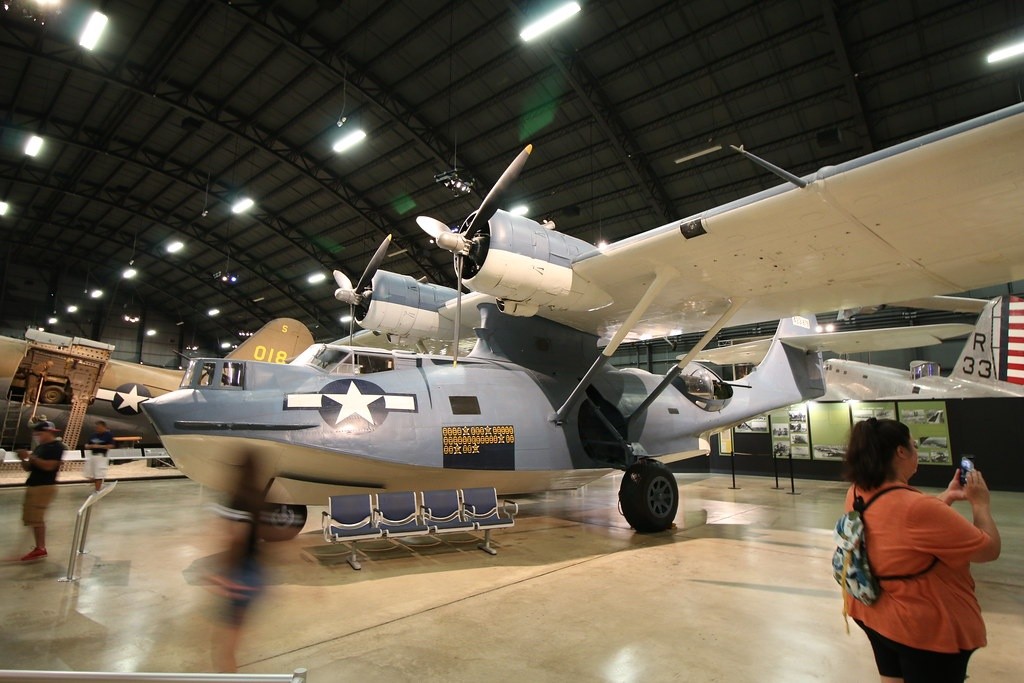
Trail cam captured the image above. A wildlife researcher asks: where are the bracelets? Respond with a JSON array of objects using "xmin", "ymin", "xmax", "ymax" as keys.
[{"xmin": 23, "ymin": 458, "xmax": 30, "ymax": 463}]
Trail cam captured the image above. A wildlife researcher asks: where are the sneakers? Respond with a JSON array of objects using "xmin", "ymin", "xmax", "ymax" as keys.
[{"xmin": 21, "ymin": 546, "xmax": 47, "ymax": 561}]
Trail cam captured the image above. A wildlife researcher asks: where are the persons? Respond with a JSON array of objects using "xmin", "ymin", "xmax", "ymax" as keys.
[
  {"xmin": 845, "ymin": 419, "xmax": 1002, "ymax": 683},
  {"xmin": 18, "ymin": 414, "xmax": 63, "ymax": 562},
  {"xmin": 85, "ymin": 419, "xmax": 113, "ymax": 493}
]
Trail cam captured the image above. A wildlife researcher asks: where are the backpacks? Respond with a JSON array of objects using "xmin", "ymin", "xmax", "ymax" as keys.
[{"xmin": 832, "ymin": 483, "xmax": 939, "ymax": 635}]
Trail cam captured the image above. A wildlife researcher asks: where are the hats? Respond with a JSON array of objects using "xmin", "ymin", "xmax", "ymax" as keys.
[
  {"xmin": 32, "ymin": 421, "xmax": 60, "ymax": 431},
  {"xmin": 38, "ymin": 414, "xmax": 47, "ymax": 420}
]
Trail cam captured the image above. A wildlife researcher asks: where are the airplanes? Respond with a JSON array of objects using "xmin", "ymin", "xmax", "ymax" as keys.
[
  {"xmin": 140, "ymin": 102, "xmax": 1024, "ymax": 535},
  {"xmin": 823, "ymin": 295, "xmax": 1024, "ymax": 401},
  {"xmin": 0, "ymin": 318, "xmax": 315, "ymax": 462}
]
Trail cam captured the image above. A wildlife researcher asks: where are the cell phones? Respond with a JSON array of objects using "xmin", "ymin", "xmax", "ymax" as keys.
[{"xmin": 960, "ymin": 457, "xmax": 974, "ymax": 486}]
